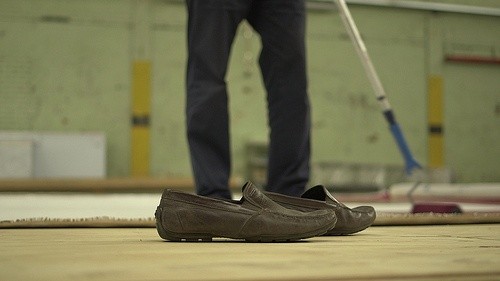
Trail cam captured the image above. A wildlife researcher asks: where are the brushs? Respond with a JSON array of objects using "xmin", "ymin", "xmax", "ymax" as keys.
[{"xmin": 335, "ymin": 0, "xmax": 500, "ymax": 203}]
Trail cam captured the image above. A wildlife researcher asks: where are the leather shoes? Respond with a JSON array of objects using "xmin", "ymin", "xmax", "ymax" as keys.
[
  {"xmin": 155, "ymin": 180, "xmax": 338, "ymax": 243},
  {"xmin": 263, "ymin": 185, "xmax": 376, "ymax": 236}
]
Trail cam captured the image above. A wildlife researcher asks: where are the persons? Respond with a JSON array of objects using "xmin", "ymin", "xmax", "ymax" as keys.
[{"xmin": 183, "ymin": 0, "xmax": 323, "ymax": 208}]
